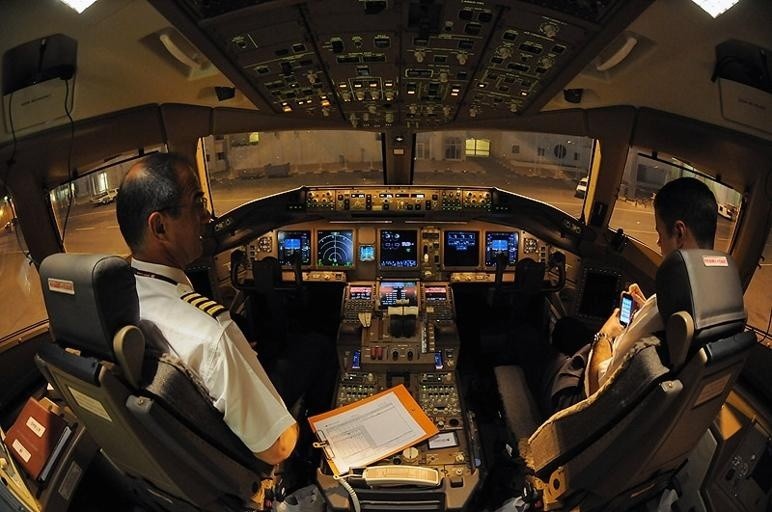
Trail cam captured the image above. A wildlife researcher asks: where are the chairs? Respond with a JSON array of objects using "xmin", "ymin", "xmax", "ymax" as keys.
[
  {"xmin": 25, "ymin": 244, "xmax": 302, "ymax": 507},
  {"xmin": 486, "ymin": 244, "xmax": 762, "ymax": 512}
]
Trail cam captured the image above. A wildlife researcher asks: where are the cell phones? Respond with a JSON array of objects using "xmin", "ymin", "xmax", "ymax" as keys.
[{"xmin": 620, "ymin": 293, "xmax": 633, "ymax": 327}]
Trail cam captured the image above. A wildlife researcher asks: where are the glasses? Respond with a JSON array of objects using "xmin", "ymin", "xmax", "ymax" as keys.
[{"xmin": 146, "ymin": 197, "xmax": 208, "ymax": 226}]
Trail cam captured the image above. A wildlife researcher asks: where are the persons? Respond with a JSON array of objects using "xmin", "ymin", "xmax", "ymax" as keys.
[
  {"xmin": 534, "ymin": 176, "xmax": 719, "ymax": 423},
  {"xmin": 115, "ymin": 151, "xmax": 301, "ymax": 466}
]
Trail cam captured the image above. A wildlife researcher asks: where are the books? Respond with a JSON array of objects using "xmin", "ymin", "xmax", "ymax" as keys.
[{"xmin": 4, "ymin": 397, "xmax": 79, "ymax": 490}]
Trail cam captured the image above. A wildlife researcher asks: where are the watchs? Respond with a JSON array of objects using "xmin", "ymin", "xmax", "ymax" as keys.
[{"xmin": 590, "ymin": 332, "xmax": 614, "ymax": 357}]
